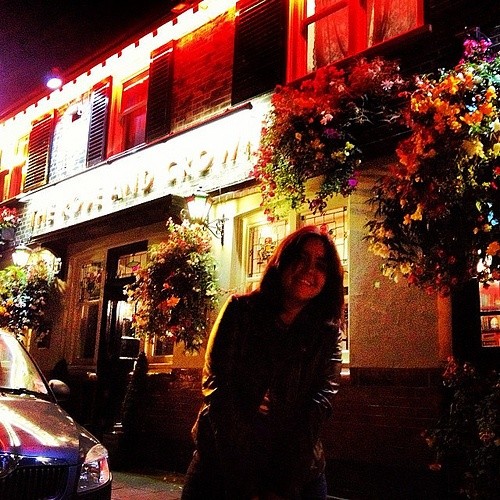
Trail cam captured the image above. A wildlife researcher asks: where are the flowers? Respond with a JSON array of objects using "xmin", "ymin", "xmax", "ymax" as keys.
[
  {"xmin": 121, "ymin": 214, "xmax": 224, "ymax": 353},
  {"xmin": 0, "ymin": 262, "xmax": 56, "ymax": 334},
  {"xmin": 249, "ymin": 37, "xmax": 500, "ymax": 297}
]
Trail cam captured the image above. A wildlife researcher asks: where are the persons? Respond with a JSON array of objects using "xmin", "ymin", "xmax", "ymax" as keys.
[{"xmin": 180, "ymin": 224, "xmax": 344, "ymax": 500}]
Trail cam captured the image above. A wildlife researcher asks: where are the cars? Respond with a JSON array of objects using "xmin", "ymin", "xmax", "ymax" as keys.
[{"xmin": 0, "ymin": 328, "xmax": 115, "ymax": 499}]
